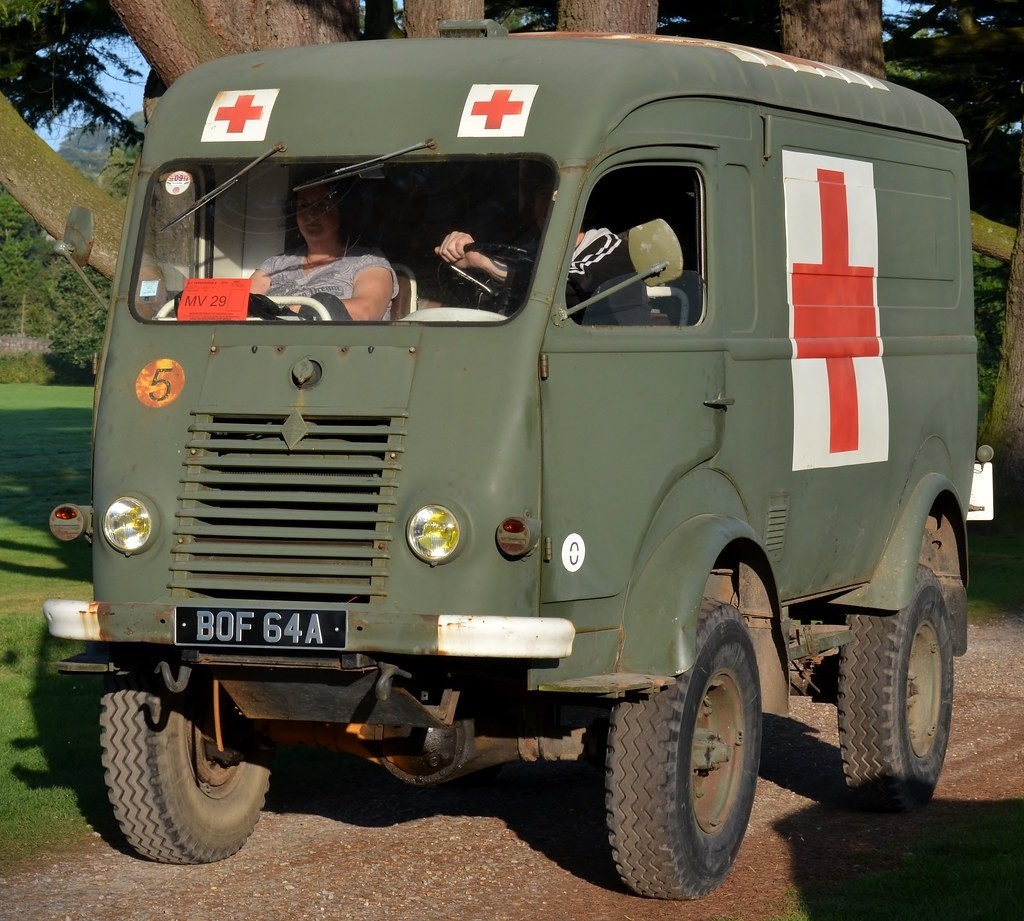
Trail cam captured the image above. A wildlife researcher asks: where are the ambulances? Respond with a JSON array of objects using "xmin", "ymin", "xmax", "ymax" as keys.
[{"xmin": 37, "ymin": 30, "xmax": 996, "ymax": 902}]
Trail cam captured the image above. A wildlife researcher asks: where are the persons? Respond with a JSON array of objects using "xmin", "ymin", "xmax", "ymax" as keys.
[
  {"xmin": 246, "ymin": 174, "xmax": 399, "ymax": 323},
  {"xmin": 435, "ymin": 175, "xmax": 649, "ymax": 327}
]
